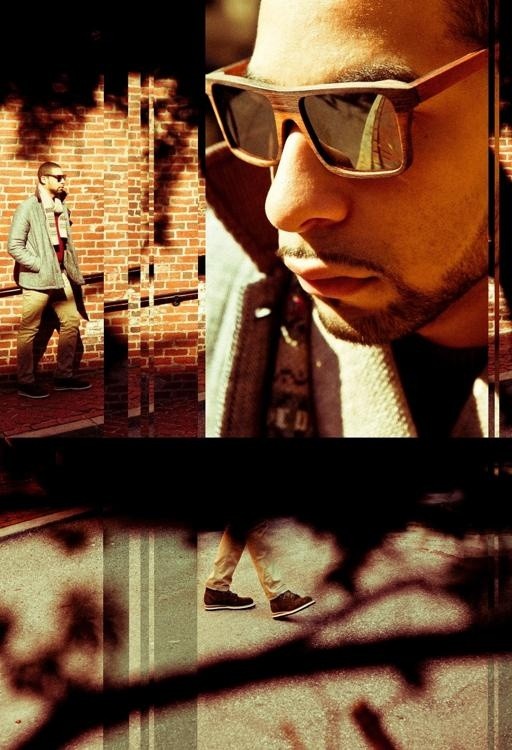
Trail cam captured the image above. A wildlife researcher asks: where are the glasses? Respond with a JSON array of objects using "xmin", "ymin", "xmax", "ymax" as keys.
[
  {"xmin": 204, "ymin": 44, "xmax": 488, "ymax": 184},
  {"xmin": 43, "ymin": 173, "xmax": 68, "ymax": 183}
]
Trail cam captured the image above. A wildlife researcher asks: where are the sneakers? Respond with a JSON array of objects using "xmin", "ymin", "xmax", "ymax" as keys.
[
  {"xmin": 53, "ymin": 379, "xmax": 92, "ymax": 391},
  {"xmin": 269, "ymin": 588, "xmax": 318, "ymax": 620},
  {"xmin": 204, "ymin": 585, "xmax": 258, "ymax": 611},
  {"xmin": 16, "ymin": 379, "xmax": 50, "ymax": 399}
]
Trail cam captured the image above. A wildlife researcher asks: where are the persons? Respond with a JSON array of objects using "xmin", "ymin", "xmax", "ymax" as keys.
[
  {"xmin": 7, "ymin": 161, "xmax": 93, "ymax": 399},
  {"xmin": 206, "ymin": 0, "xmax": 499, "ymax": 440},
  {"xmin": 201, "ymin": 522, "xmax": 316, "ymax": 619}
]
